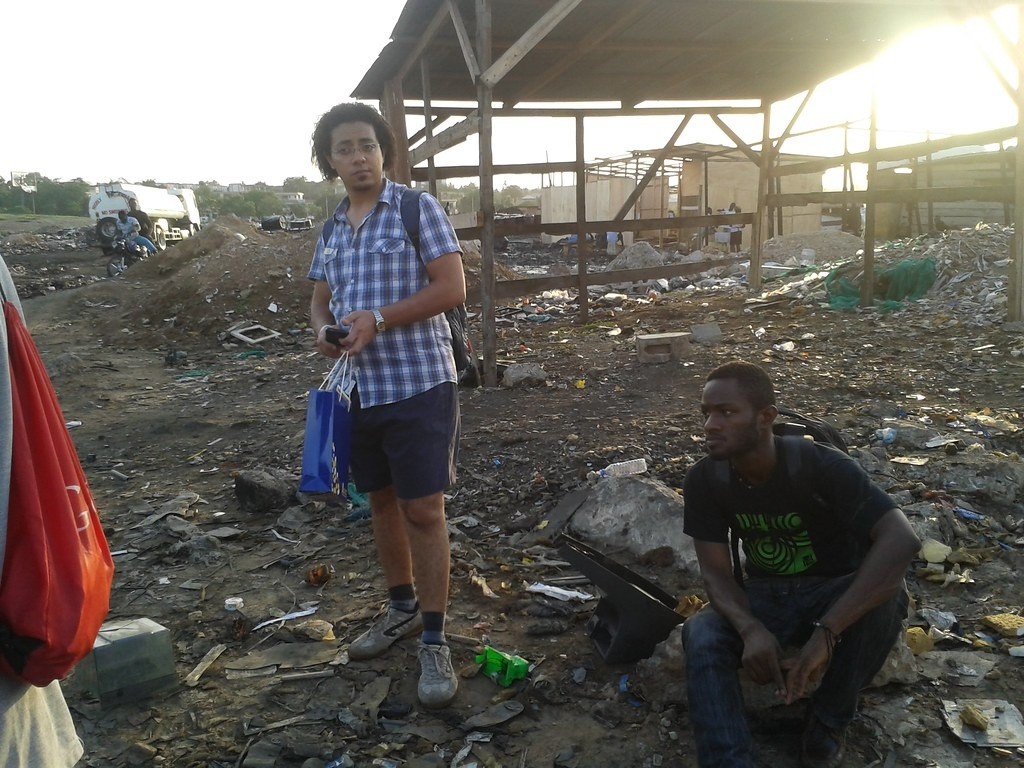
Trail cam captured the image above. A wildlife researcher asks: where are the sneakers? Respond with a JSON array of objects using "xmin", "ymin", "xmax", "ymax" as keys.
[
  {"xmin": 348, "ymin": 601, "xmax": 423, "ymax": 661},
  {"xmin": 416, "ymin": 639, "xmax": 458, "ymax": 709}
]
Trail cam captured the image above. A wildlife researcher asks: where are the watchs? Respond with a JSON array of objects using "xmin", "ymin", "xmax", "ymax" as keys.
[{"xmin": 373, "ymin": 309, "xmax": 386, "ymax": 331}]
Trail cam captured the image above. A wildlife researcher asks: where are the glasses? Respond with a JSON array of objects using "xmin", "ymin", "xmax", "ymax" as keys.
[{"xmin": 328, "ymin": 142, "xmax": 381, "ymax": 161}]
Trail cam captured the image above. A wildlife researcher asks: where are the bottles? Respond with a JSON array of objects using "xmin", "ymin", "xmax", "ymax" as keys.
[{"xmin": 600, "ymin": 458, "xmax": 647, "ymax": 477}]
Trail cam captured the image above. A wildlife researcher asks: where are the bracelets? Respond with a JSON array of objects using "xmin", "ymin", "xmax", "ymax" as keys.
[{"xmin": 813, "ymin": 620, "xmax": 842, "ymax": 661}]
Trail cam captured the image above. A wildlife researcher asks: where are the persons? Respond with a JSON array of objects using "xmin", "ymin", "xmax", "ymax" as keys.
[
  {"xmin": 305, "ymin": 102, "xmax": 469, "ymax": 708},
  {"xmin": 117, "ymin": 211, "xmax": 156, "ymax": 255},
  {"xmin": 127, "ymin": 198, "xmax": 163, "ymax": 251},
  {"xmin": 682, "ymin": 362, "xmax": 923, "ymax": 767},
  {"xmin": 706, "ymin": 201, "xmax": 744, "ymax": 253},
  {"xmin": 0, "ymin": 249, "xmax": 86, "ymax": 768}
]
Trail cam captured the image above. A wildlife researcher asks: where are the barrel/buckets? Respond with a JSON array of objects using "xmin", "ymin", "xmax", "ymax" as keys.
[{"xmin": 801, "ymin": 249, "xmax": 815, "ymax": 265}]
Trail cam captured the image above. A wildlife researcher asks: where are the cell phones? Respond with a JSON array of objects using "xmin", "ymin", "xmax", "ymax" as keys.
[{"xmin": 326, "ymin": 327, "xmax": 350, "ymax": 346}]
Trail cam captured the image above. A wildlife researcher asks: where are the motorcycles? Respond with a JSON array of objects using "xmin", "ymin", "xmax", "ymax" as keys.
[{"xmin": 106, "ymin": 236, "xmax": 142, "ymax": 276}]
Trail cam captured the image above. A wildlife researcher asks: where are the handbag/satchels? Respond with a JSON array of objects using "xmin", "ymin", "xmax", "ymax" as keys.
[
  {"xmin": 3, "ymin": 300, "xmax": 115, "ymax": 687},
  {"xmin": 298, "ymin": 349, "xmax": 352, "ymax": 503}
]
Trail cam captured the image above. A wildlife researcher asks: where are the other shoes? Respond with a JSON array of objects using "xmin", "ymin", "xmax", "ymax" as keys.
[{"xmin": 801, "ymin": 702, "xmax": 845, "ymax": 768}]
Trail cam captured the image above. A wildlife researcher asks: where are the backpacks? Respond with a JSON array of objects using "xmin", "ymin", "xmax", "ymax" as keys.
[
  {"xmin": 323, "ymin": 188, "xmax": 473, "ymax": 381},
  {"xmin": 702, "ymin": 409, "xmax": 858, "ymax": 576}
]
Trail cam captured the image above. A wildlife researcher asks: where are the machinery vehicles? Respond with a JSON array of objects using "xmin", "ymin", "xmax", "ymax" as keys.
[{"xmin": 87, "ymin": 181, "xmax": 202, "ymax": 252}]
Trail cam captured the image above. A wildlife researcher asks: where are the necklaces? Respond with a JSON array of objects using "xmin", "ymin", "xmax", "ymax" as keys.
[{"xmin": 731, "ymin": 465, "xmax": 753, "ymax": 490}]
